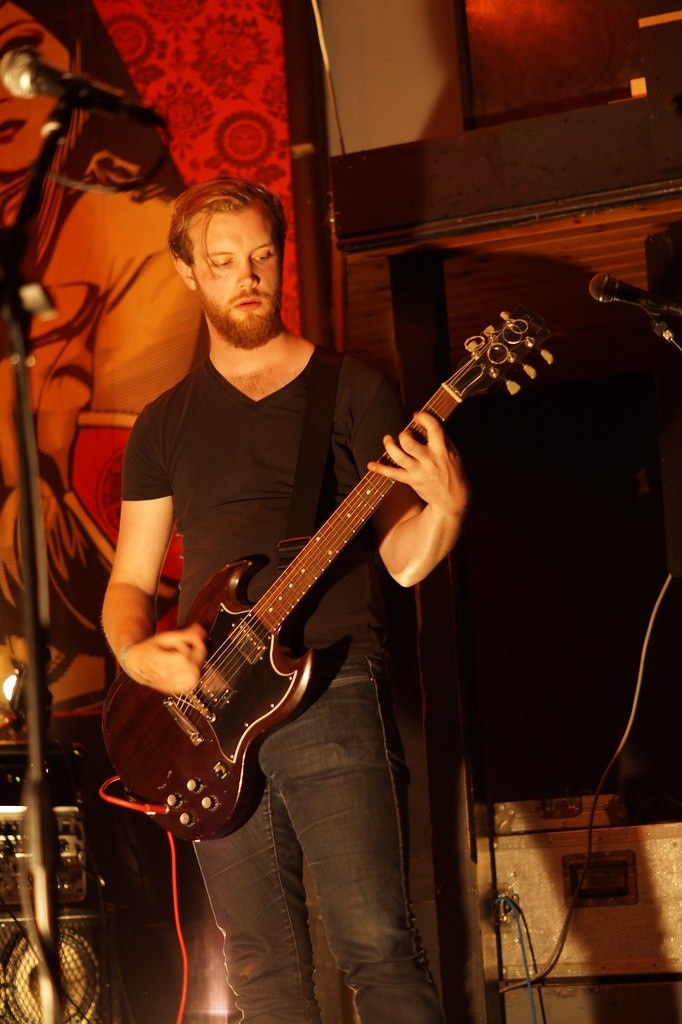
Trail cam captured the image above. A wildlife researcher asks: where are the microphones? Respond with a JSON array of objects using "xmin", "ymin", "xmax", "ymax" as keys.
[
  {"xmin": 0, "ymin": 49, "xmax": 166, "ymax": 128},
  {"xmin": 588, "ymin": 273, "xmax": 682, "ymax": 319}
]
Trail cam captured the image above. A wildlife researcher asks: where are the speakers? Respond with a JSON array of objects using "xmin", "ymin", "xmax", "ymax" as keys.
[
  {"xmin": 442, "ymin": 370, "xmax": 681, "ymax": 802},
  {"xmin": 0, "ymin": 905, "xmax": 122, "ymax": 1024}
]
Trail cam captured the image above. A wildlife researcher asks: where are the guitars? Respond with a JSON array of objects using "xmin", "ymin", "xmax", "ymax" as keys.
[{"xmin": 101, "ymin": 302, "xmax": 554, "ymax": 844}]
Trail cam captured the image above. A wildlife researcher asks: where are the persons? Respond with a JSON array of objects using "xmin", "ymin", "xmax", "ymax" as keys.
[{"xmin": 99, "ymin": 176, "xmax": 469, "ymax": 1024}]
